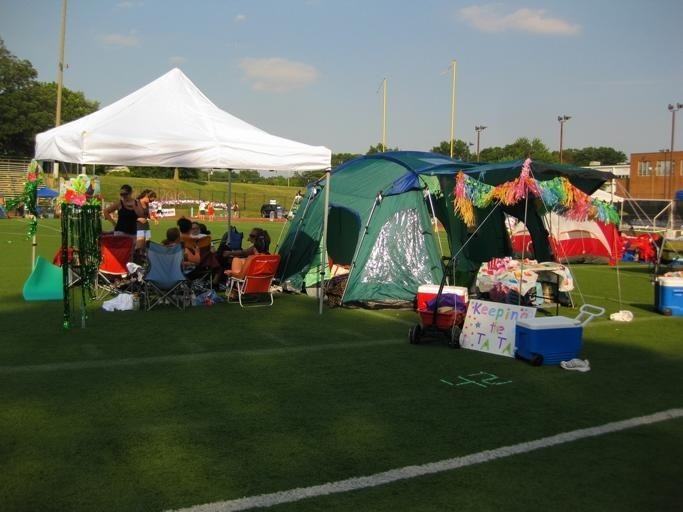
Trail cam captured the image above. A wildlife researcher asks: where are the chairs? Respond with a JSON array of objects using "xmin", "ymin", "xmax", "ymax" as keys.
[
  {"xmin": 141, "ymin": 241, "xmax": 190, "ymax": 313},
  {"xmin": 97, "ymin": 232, "xmax": 137, "ymax": 302},
  {"xmin": 226, "ymin": 253, "xmax": 282, "ymax": 309},
  {"xmin": 209, "ymin": 230, "xmax": 243, "ymax": 267}
]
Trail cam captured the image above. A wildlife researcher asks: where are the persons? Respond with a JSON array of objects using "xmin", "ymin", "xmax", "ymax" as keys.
[
  {"xmin": 150, "ymin": 199, "xmax": 271, "ymax": 289},
  {"xmin": 134, "ymin": 190, "xmax": 159, "ymax": 265},
  {"xmin": 104, "ymin": 185, "xmax": 145, "ymax": 281},
  {"xmin": 0, "ymin": 196, "xmax": 8, "ymax": 218}
]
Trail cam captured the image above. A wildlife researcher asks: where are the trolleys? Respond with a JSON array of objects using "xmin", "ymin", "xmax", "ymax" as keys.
[{"xmin": 409, "ymin": 256, "xmax": 470, "ymax": 349}]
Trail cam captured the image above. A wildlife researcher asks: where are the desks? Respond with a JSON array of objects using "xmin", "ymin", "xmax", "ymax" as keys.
[{"xmin": 477, "ymin": 260, "xmax": 561, "ymax": 316}]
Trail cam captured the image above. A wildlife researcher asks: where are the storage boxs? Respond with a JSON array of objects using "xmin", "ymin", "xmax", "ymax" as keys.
[
  {"xmin": 650, "ymin": 275, "xmax": 683, "ymax": 318},
  {"xmin": 516, "ymin": 312, "xmax": 585, "ymax": 367},
  {"xmin": 417, "ymin": 286, "xmax": 468, "ymax": 313}
]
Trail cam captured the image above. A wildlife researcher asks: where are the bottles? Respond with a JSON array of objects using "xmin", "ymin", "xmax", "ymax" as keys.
[
  {"xmin": 269, "ymin": 210, "xmax": 274, "ymax": 221},
  {"xmin": 132, "ymin": 290, "xmax": 141, "ymax": 311}
]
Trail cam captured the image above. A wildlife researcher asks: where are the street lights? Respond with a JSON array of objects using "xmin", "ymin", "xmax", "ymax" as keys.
[
  {"xmin": 557, "ymin": 114, "xmax": 572, "ymax": 166},
  {"xmin": 667, "ymin": 101, "xmax": 683, "ymax": 200},
  {"xmin": 474, "ymin": 125, "xmax": 488, "ymax": 164}
]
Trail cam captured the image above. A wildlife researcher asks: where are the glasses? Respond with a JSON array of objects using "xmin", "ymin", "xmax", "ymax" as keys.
[
  {"xmin": 148, "ymin": 199, "xmax": 152, "ymax": 203},
  {"xmin": 249, "ymin": 233, "xmax": 255, "ymax": 238},
  {"xmin": 120, "ymin": 192, "xmax": 128, "ymax": 196}
]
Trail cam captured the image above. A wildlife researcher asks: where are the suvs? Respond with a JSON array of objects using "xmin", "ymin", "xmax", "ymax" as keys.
[{"xmin": 261, "ymin": 204, "xmax": 287, "ymax": 218}]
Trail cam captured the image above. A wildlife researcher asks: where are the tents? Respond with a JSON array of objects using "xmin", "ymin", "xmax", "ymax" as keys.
[
  {"xmin": 510, "ymin": 210, "xmax": 624, "ymax": 263},
  {"xmin": 273, "ymin": 150, "xmax": 577, "ymax": 306}
]
